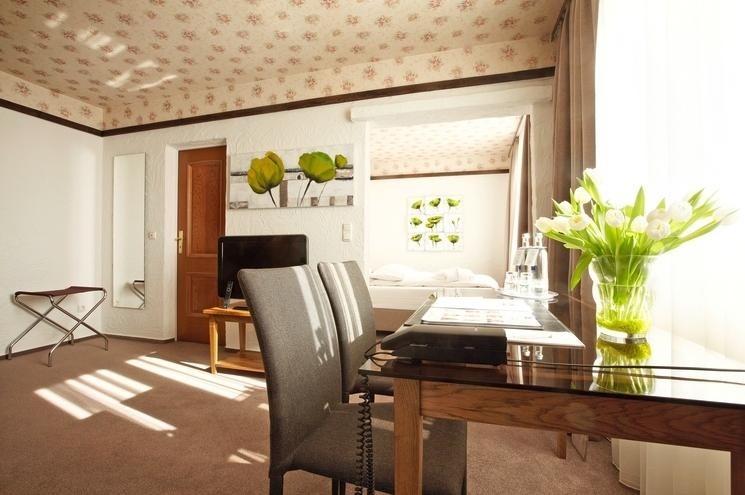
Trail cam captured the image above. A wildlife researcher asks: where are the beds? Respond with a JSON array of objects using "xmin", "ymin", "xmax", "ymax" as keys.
[{"xmin": 369, "ymin": 269, "xmax": 499, "ymax": 333}]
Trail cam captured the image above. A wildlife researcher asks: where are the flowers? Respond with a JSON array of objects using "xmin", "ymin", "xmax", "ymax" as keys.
[{"xmin": 536, "ymin": 165, "xmax": 737, "ymax": 336}]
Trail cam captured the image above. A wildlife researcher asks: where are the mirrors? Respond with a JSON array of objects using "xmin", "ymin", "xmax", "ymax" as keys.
[{"xmin": 113, "ymin": 152, "xmax": 145, "ymax": 309}]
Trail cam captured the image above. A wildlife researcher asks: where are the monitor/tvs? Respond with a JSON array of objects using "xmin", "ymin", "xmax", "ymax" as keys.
[{"xmin": 217, "ymin": 234, "xmax": 309, "ymax": 311}]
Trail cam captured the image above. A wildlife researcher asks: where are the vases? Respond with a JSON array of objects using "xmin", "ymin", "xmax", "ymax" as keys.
[{"xmin": 589, "ymin": 254, "xmax": 657, "ymax": 343}]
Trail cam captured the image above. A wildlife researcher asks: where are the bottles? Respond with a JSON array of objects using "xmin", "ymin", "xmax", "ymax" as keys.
[{"xmin": 514, "ymin": 233, "xmax": 549, "ymax": 294}]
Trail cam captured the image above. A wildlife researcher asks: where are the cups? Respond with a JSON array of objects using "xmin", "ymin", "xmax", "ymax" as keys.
[
  {"xmin": 310, "ymin": 195, "xmax": 353, "ymax": 206},
  {"xmin": 504, "ymin": 271, "xmax": 533, "ymax": 294}
]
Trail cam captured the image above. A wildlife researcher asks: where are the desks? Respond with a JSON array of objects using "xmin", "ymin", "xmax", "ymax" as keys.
[
  {"xmin": 202, "ymin": 301, "xmax": 265, "ymax": 374},
  {"xmin": 357, "ymin": 284, "xmax": 745, "ymax": 493}
]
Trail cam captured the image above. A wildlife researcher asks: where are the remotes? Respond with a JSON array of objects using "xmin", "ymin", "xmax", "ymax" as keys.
[{"xmin": 222, "ymin": 281, "xmax": 234, "ymax": 310}]
